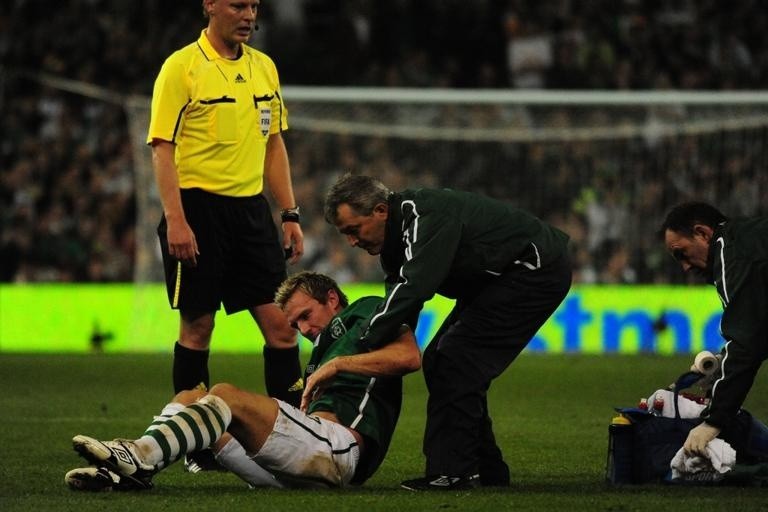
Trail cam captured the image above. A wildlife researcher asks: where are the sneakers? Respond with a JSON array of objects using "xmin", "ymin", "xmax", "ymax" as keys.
[
  {"xmin": 401, "ymin": 459, "xmax": 510, "ymax": 492},
  {"xmin": 73, "ymin": 435, "xmax": 154, "ymax": 489},
  {"xmin": 65, "ymin": 468, "xmax": 154, "ymax": 492},
  {"xmin": 184, "ymin": 454, "xmax": 202, "ymax": 473}
]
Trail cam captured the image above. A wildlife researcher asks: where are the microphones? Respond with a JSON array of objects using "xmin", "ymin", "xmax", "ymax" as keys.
[{"xmin": 255, "ymin": 20, "xmax": 259, "ymax": 31}]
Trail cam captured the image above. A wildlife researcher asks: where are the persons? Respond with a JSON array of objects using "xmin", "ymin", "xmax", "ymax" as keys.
[
  {"xmin": 145, "ymin": 0, "xmax": 304, "ymax": 470},
  {"xmin": 661, "ymin": 202, "xmax": 767, "ymax": 456},
  {"xmin": 0, "ymin": 1, "xmax": 768, "ymax": 283},
  {"xmin": 325, "ymin": 172, "xmax": 573, "ymax": 490},
  {"xmin": 65, "ymin": 271, "xmax": 420, "ymax": 492}
]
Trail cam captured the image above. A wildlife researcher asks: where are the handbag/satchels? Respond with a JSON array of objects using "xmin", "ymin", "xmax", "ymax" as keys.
[{"xmin": 615, "ymin": 371, "xmax": 768, "ymax": 484}]
